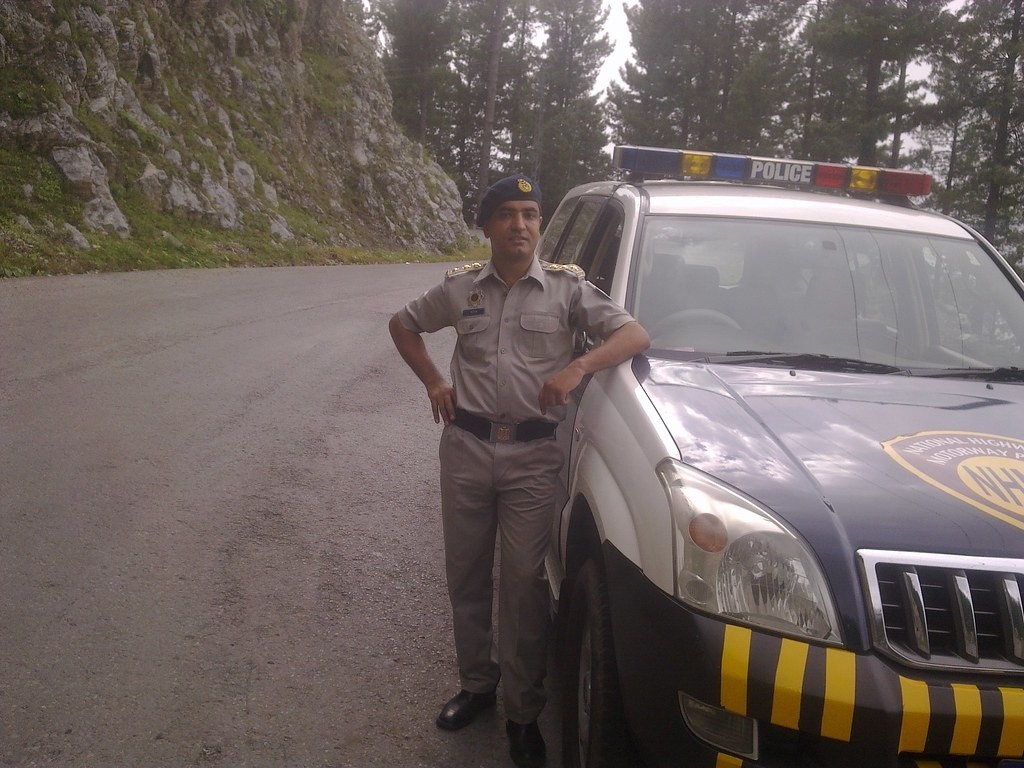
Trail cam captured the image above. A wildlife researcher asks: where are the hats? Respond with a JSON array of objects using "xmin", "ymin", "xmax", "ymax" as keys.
[{"xmin": 477, "ymin": 174, "xmax": 542, "ymax": 227}]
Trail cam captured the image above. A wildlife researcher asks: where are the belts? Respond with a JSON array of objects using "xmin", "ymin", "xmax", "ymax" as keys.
[{"xmin": 454, "ymin": 408, "xmax": 554, "ymax": 442}]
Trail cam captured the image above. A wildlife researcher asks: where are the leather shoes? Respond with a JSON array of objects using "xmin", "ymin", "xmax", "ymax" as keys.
[
  {"xmin": 436, "ymin": 689, "xmax": 496, "ymax": 730},
  {"xmin": 506, "ymin": 719, "xmax": 546, "ymax": 768}
]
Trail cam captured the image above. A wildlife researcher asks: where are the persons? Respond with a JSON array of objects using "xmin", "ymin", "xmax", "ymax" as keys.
[{"xmin": 388, "ymin": 173, "xmax": 651, "ymax": 768}]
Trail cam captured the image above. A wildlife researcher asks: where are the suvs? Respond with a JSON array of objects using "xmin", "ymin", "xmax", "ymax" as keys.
[{"xmin": 536, "ymin": 144, "xmax": 1024, "ymax": 767}]
